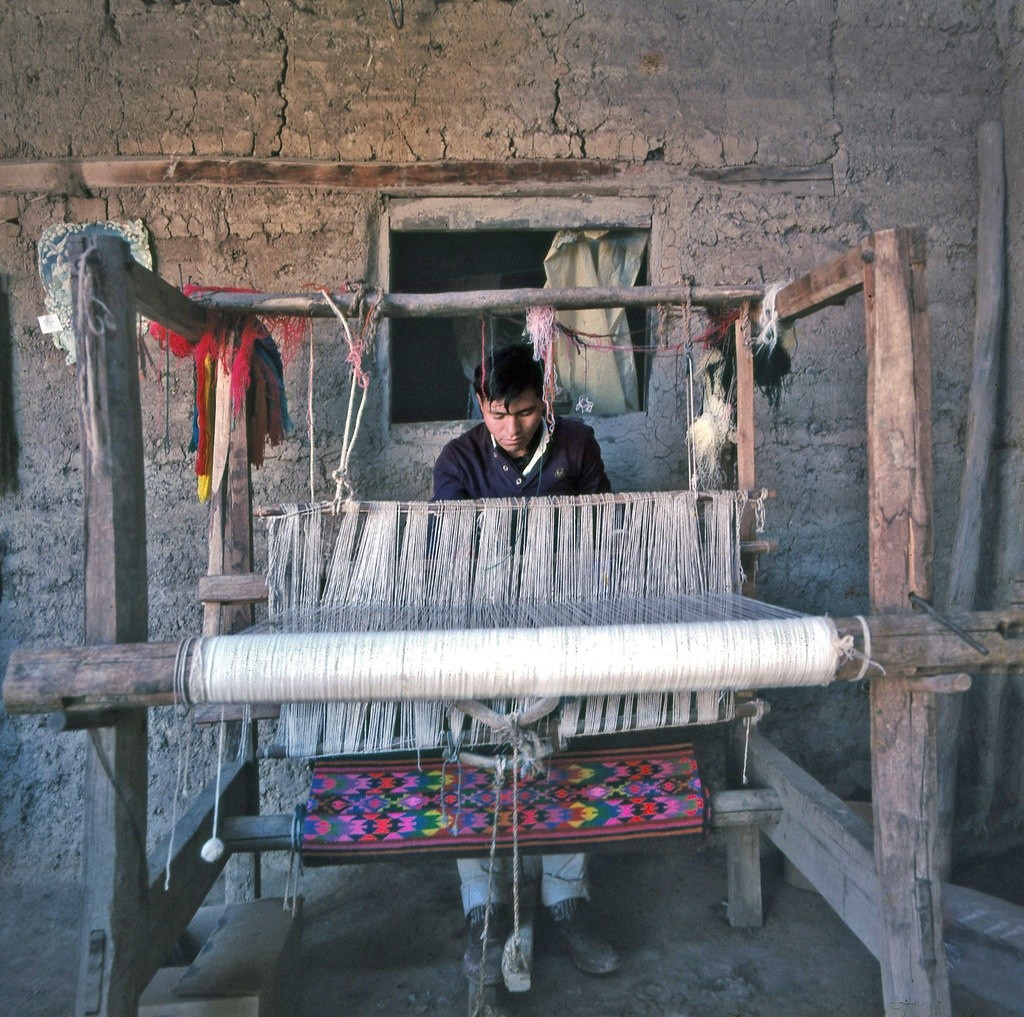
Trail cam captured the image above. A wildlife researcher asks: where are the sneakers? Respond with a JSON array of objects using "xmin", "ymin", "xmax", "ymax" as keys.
[
  {"xmin": 545, "ymin": 897, "xmax": 621, "ymax": 974},
  {"xmin": 462, "ymin": 902, "xmax": 505, "ymax": 985}
]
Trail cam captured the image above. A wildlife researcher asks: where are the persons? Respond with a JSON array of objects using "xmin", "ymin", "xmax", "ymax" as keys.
[{"xmin": 428, "ymin": 345, "xmax": 619, "ymax": 985}]
